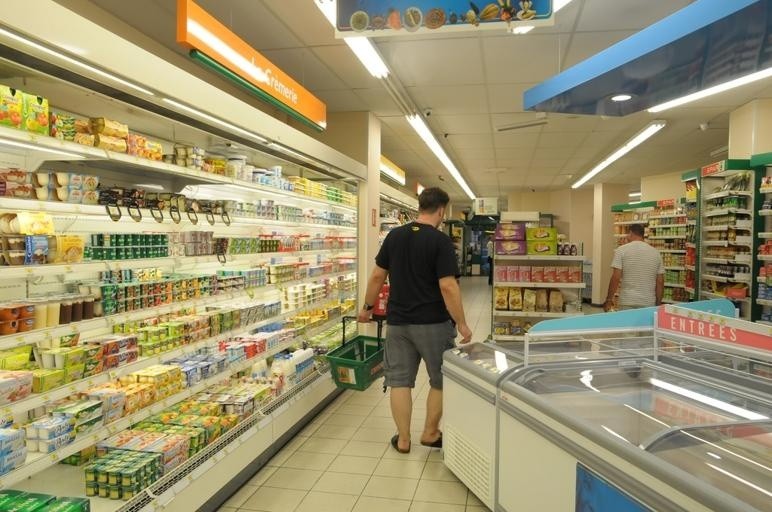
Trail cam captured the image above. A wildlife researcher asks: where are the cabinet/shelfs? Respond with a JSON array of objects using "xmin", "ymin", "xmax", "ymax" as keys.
[
  {"xmin": 492, "ymin": 240, "xmax": 587, "ymax": 341},
  {"xmin": 611, "ymin": 152, "xmax": 772, "ymax": 324},
  {"xmin": 380, "ymin": 183, "xmax": 420, "ymax": 245},
  {"xmin": 0, "ymin": 1, "xmax": 368, "ymax": 512}
]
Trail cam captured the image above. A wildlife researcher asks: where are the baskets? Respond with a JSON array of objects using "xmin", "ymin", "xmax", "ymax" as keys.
[{"xmin": 324, "ymin": 315, "xmax": 387, "ymax": 392}]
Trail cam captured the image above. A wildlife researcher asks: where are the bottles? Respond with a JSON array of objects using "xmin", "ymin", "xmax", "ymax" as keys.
[
  {"xmin": 269, "ymin": 348, "xmax": 314, "ymax": 385},
  {"xmin": 564, "ymin": 243, "xmax": 570, "ymax": 255},
  {"xmin": 648, "ymin": 217, "xmax": 687, "ymax": 299},
  {"xmin": 570, "ymin": 243, "xmax": 577, "ymax": 256},
  {"xmin": 557, "ymin": 243, "xmax": 563, "ymax": 255},
  {"xmin": 253, "ymin": 360, "xmax": 269, "ymax": 380}
]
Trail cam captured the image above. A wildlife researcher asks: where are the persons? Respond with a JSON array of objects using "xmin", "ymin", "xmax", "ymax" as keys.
[
  {"xmin": 357, "ymin": 187, "xmax": 471, "ymax": 452},
  {"xmin": 603, "ymin": 225, "xmax": 665, "ymax": 338}
]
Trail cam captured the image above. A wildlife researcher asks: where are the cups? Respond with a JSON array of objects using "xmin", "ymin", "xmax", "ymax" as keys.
[{"xmin": 20, "ymin": 294, "xmax": 96, "ymax": 328}]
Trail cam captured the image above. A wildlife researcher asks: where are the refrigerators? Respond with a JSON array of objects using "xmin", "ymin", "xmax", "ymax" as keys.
[
  {"xmin": 497, "ymin": 353, "xmax": 772, "ymax": 512},
  {"xmin": 442, "ymin": 327, "xmax": 754, "ymax": 511}
]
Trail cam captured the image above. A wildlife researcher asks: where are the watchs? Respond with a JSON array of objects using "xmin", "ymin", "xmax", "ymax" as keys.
[{"xmin": 361, "ymin": 304, "xmax": 376, "ymax": 311}]
